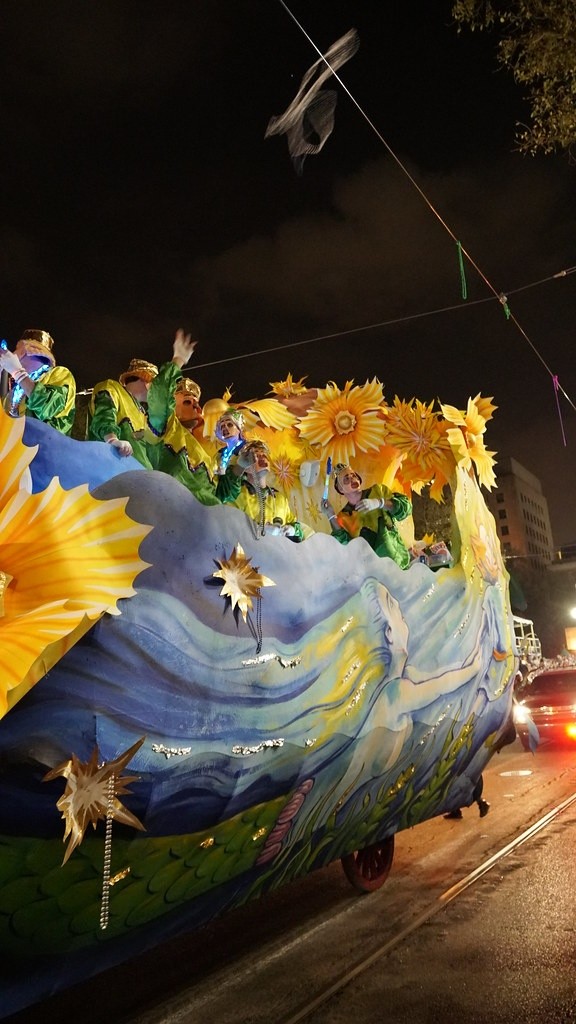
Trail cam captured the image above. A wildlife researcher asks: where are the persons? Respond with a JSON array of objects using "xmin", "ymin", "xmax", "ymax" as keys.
[
  {"xmin": 212, "ymin": 407, "xmax": 249, "ymax": 486},
  {"xmin": 443, "ymin": 774, "xmax": 490, "ymax": 820},
  {"xmin": 321, "ymin": 462, "xmax": 413, "ymax": 570},
  {"xmin": 89, "ymin": 328, "xmax": 222, "ymax": 506},
  {"xmin": 216, "ymin": 439, "xmax": 316, "ymax": 543},
  {"xmin": 0, "ymin": 329, "xmax": 75, "ymax": 435}
]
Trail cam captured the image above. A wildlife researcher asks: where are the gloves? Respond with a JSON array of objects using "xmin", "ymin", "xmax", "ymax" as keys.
[
  {"xmin": 355, "ymin": 498, "xmax": 385, "ymax": 514},
  {"xmin": 321, "ymin": 498, "xmax": 337, "ymax": 521}
]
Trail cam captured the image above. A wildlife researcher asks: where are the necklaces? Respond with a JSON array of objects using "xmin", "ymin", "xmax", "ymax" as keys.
[
  {"xmin": 126, "ymin": 389, "xmax": 146, "ymax": 414},
  {"xmin": 9, "ymin": 365, "xmax": 49, "ymax": 417},
  {"xmin": 249, "ymin": 452, "xmax": 266, "ymax": 540}
]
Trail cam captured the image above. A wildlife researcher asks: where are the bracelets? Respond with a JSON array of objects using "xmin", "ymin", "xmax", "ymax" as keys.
[
  {"xmin": 107, "ymin": 438, "xmax": 118, "ymax": 443},
  {"xmin": 380, "ymin": 498, "xmax": 384, "ymax": 508},
  {"xmin": 15, "ymin": 372, "xmax": 27, "ymax": 382},
  {"xmin": 12, "ymin": 368, "xmax": 25, "ymax": 376},
  {"xmin": 18, "ymin": 374, "xmax": 29, "ymax": 383},
  {"xmin": 329, "ymin": 515, "xmax": 337, "ymax": 520}
]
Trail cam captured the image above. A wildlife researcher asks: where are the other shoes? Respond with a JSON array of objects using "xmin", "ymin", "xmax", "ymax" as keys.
[
  {"xmin": 443, "ymin": 810, "xmax": 463, "ymax": 818},
  {"xmin": 479, "ymin": 802, "xmax": 490, "ymax": 817}
]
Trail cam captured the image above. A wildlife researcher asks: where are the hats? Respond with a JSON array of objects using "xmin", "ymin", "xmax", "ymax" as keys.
[
  {"xmin": 119, "ymin": 358, "xmax": 158, "ymax": 390},
  {"xmin": 174, "ymin": 379, "xmax": 201, "ymax": 399},
  {"xmin": 220, "ymin": 408, "xmax": 243, "ymax": 432},
  {"xmin": 13, "ymin": 329, "xmax": 57, "ymax": 367}
]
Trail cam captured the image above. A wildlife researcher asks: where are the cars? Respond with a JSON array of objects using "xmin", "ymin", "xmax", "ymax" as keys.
[{"xmin": 513, "ymin": 668, "xmax": 576, "ymax": 751}]
